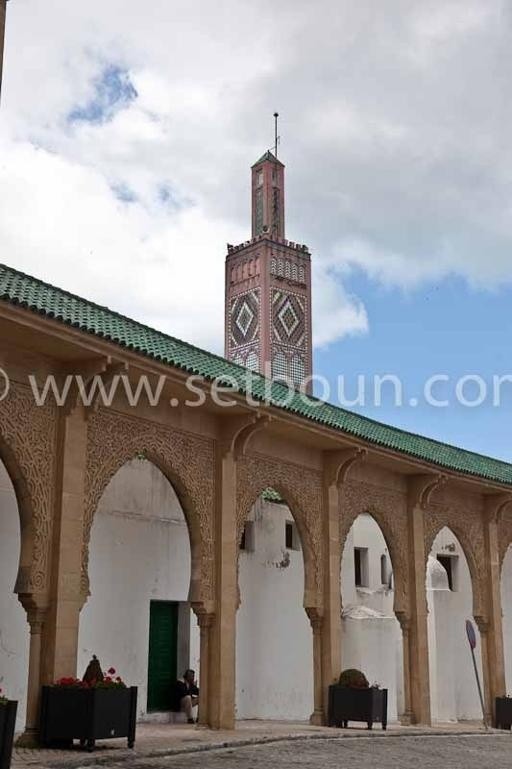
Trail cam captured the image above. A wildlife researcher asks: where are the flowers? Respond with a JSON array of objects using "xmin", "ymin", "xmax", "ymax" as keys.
[
  {"xmin": 331, "ymin": 677, "xmax": 383, "ymax": 689},
  {"xmin": 1, "ymin": 687, "xmax": 9, "ymax": 701},
  {"xmin": 48, "ymin": 663, "xmax": 127, "ymax": 690}
]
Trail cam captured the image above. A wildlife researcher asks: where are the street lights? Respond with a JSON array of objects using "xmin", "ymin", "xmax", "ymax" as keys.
[{"xmin": 274, "ymin": 112, "xmax": 279, "ymax": 159}]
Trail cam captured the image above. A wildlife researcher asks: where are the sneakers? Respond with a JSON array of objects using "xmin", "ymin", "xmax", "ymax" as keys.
[{"xmin": 186, "ymin": 717, "xmax": 199, "ymax": 724}]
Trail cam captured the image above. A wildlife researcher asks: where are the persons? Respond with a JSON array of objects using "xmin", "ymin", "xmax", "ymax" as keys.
[{"xmin": 170, "ymin": 667, "xmax": 200, "ymax": 724}]
